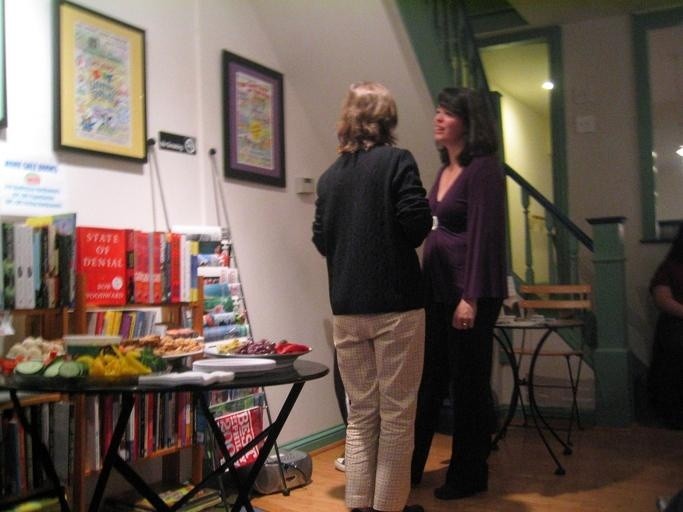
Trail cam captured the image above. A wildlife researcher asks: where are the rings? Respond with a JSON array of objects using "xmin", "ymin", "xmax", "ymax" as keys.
[{"xmin": 463, "ymin": 323, "xmax": 467, "ymax": 326}]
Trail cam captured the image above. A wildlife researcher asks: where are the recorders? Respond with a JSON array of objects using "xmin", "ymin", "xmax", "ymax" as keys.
[{"xmin": 252, "ymin": 449, "xmax": 313, "ymax": 496}]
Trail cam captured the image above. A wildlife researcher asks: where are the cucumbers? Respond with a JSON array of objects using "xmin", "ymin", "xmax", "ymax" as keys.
[{"xmin": 14, "ymin": 357, "xmax": 88, "ymax": 378}]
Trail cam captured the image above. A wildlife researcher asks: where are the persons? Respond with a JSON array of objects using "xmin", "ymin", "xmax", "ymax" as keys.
[
  {"xmin": 649, "ymin": 221, "xmax": 683, "ymax": 429},
  {"xmin": 411, "ymin": 86, "xmax": 508, "ymax": 501},
  {"xmin": 311, "ymin": 82, "xmax": 433, "ymax": 512},
  {"xmin": 333, "ymin": 355, "xmax": 348, "ymax": 471}
]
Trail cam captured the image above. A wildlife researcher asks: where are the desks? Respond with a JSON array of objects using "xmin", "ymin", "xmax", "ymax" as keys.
[
  {"xmin": 0, "ymin": 360, "xmax": 329, "ymax": 512},
  {"xmin": 491, "ymin": 315, "xmax": 589, "ymax": 480}
]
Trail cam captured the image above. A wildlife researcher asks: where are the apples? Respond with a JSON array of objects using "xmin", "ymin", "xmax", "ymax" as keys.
[{"xmin": 95, "ymin": 355, "xmax": 116, "ymax": 376}]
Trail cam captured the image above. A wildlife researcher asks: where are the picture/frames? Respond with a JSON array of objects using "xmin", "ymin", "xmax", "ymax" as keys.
[
  {"xmin": 52, "ymin": 0, "xmax": 149, "ymax": 165},
  {"xmin": 0, "ymin": 0, "xmax": 7, "ymax": 127},
  {"xmin": 221, "ymin": 49, "xmax": 286, "ymax": 187}
]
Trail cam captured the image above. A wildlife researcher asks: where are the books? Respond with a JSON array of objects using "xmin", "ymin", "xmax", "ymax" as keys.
[{"xmin": 1, "ymin": 212, "xmax": 264, "ymax": 511}]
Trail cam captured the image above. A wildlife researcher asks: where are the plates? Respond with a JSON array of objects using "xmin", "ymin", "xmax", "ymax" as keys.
[{"xmin": 161, "ymin": 336, "xmax": 312, "ymax": 378}]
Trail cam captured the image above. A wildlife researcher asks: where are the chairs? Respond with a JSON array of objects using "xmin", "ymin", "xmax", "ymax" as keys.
[
  {"xmin": 0, "ymin": 277, "xmax": 212, "ymax": 512},
  {"xmin": 512, "ymin": 283, "xmax": 595, "ymax": 447}
]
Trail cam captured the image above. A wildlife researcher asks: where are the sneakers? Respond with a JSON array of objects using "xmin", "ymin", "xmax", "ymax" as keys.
[
  {"xmin": 411, "ymin": 473, "xmax": 422, "ymax": 485},
  {"xmin": 334, "ymin": 458, "xmax": 346, "ymax": 472},
  {"xmin": 434, "ymin": 479, "xmax": 488, "ymax": 500},
  {"xmin": 402, "ymin": 505, "xmax": 425, "ymax": 512}
]
